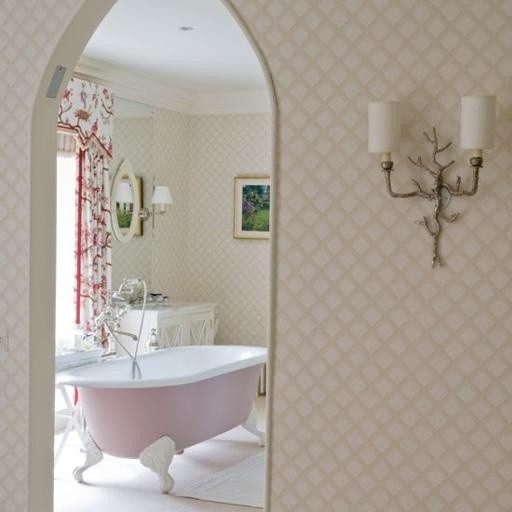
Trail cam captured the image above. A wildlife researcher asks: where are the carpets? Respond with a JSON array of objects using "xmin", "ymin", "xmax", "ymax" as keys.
[{"xmin": 175, "ymin": 451, "xmax": 266, "ymax": 508}]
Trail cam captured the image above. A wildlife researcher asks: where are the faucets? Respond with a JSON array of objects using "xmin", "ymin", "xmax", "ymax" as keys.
[{"xmin": 148, "ymin": 293, "xmax": 161, "ymax": 303}]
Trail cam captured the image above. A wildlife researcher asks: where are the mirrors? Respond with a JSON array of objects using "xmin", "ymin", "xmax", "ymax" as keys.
[{"xmin": 112, "ymin": 159, "xmax": 139, "ymax": 243}]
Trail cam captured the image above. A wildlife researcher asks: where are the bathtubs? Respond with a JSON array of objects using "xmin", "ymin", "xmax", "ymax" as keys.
[{"xmin": 54, "ymin": 344, "xmax": 268, "ymax": 494}]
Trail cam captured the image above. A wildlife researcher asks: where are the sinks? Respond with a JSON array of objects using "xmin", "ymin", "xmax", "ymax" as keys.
[{"xmin": 119, "ymin": 301, "xmax": 217, "ymax": 312}]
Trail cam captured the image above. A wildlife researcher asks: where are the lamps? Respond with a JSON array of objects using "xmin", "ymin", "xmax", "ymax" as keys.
[
  {"xmin": 367, "ymin": 95, "xmax": 495, "ymax": 269},
  {"xmin": 138, "ymin": 187, "xmax": 173, "ymax": 220},
  {"xmin": 116, "ymin": 182, "xmax": 133, "ymax": 214}
]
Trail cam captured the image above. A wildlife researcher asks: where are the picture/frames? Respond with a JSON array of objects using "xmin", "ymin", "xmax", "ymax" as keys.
[{"xmin": 234, "ymin": 177, "xmax": 270, "ymax": 240}]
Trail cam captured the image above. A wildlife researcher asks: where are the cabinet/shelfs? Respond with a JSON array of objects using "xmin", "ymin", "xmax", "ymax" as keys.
[{"xmin": 115, "ymin": 302, "xmax": 216, "ymax": 354}]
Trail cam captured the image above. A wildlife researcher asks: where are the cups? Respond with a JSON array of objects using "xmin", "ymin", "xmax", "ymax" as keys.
[{"xmin": 74, "ymin": 335, "xmax": 84, "ymax": 349}]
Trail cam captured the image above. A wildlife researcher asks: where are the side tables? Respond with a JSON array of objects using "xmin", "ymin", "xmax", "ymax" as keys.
[{"xmin": 54, "ymin": 347, "xmax": 106, "ymax": 472}]
